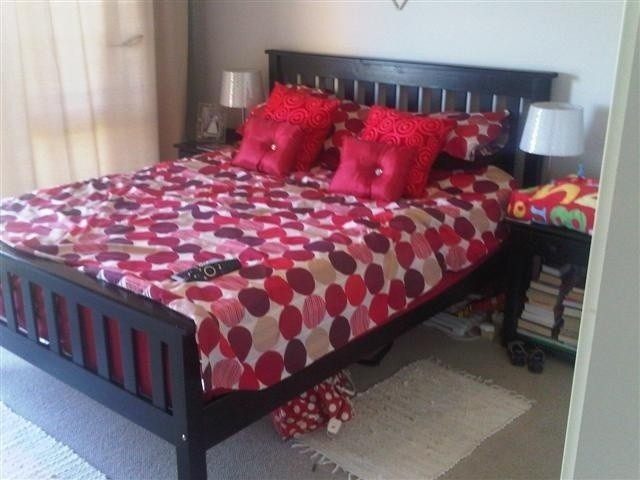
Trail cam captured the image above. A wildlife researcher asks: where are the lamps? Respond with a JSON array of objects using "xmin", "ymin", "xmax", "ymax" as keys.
[
  {"xmin": 218, "ymin": 67, "xmax": 264, "ymax": 127},
  {"xmin": 519, "ymin": 101, "xmax": 587, "ymax": 172}
]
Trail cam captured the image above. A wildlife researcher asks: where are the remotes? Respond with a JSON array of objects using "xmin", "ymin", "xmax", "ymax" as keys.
[{"xmin": 183, "ymin": 259, "xmax": 240, "ymax": 281}]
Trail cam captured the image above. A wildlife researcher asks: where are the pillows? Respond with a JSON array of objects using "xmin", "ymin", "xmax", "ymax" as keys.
[{"xmin": 234, "ymin": 82, "xmax": 511, "ymax": 208}]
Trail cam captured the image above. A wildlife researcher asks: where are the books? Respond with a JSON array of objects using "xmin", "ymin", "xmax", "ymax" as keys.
[
  {"xmin": 195, "ymin": 142, "xmax": 231, "ymax": 153},
  {"xmin": 512, "ymin": 256, "xmax": 589, "ymax": 358}
]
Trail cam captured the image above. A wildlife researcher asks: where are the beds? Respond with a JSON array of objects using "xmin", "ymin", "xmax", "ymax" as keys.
[{"xmin": 1, "ymin": 49, "xmax": 559, "ymax": 478}]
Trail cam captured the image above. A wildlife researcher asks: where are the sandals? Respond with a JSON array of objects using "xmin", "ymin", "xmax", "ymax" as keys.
[{"xmin": 507, "ymin": 339, "xmax": 546, "ymax": 373}]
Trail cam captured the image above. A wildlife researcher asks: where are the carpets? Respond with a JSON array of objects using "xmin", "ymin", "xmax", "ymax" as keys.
[
  {"xmin": 1, "ymin": 399, "xmax": 114, "ymax": 479},
  {"xmin": 295, "ymin": 350, "xmax": 536, "ymax": 480}
]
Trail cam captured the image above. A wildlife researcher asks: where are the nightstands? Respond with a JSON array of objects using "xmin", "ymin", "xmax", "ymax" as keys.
[
  {"xmin": 172, "ymin": 134, "xmax": 225, "ymax": 160},
  {"xmin": 500, "ymin": 174, "xmax": 602, "ymax": 364}
]
